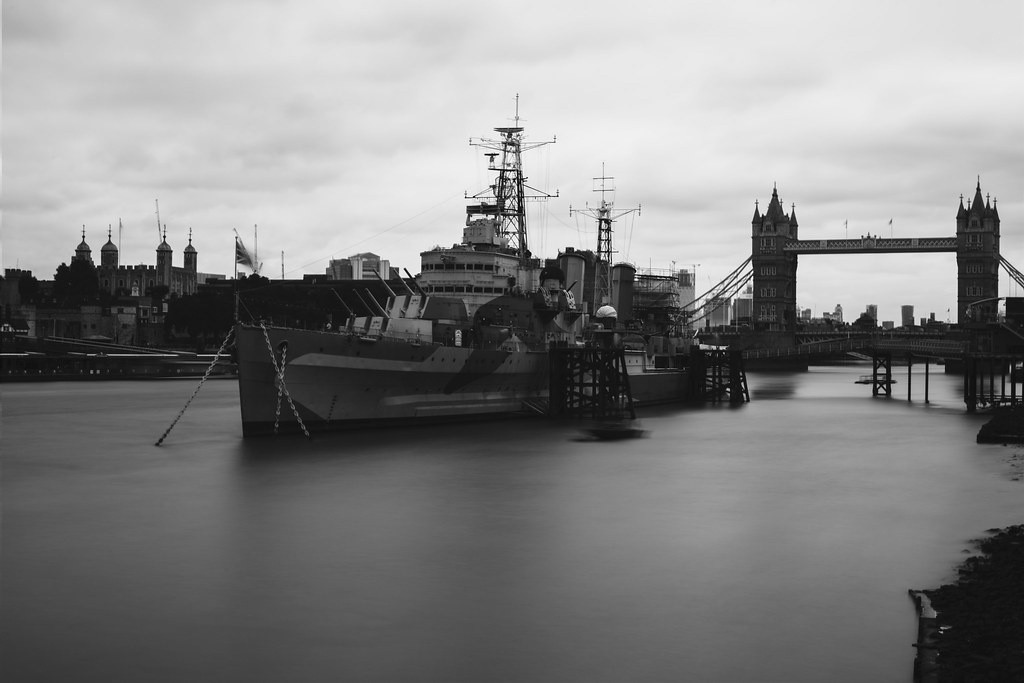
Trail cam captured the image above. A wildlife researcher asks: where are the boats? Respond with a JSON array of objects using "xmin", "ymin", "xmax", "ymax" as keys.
[{"xmin": 855, "ymin": 373, "xmax": 897, "ymax": 385}]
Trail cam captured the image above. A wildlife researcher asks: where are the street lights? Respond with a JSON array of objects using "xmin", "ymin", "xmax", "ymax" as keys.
[
  {"xmin": 114, "ymin": 311, "xmax": 117, "ymax": 343},
  {"xmin": 53, "ymin": 313, "xmax": 56, "ymax": 336}
]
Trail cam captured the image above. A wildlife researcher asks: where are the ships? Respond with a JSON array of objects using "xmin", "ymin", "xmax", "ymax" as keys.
[{"xmin": 231, "ymin": 94, "xmax": 699, "ymax": 438}]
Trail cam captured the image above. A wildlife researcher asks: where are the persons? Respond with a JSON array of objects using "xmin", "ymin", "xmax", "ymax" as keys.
[{"xmin": 327, "ymin": 321, "xmax": 332, "ymax": 332}]
